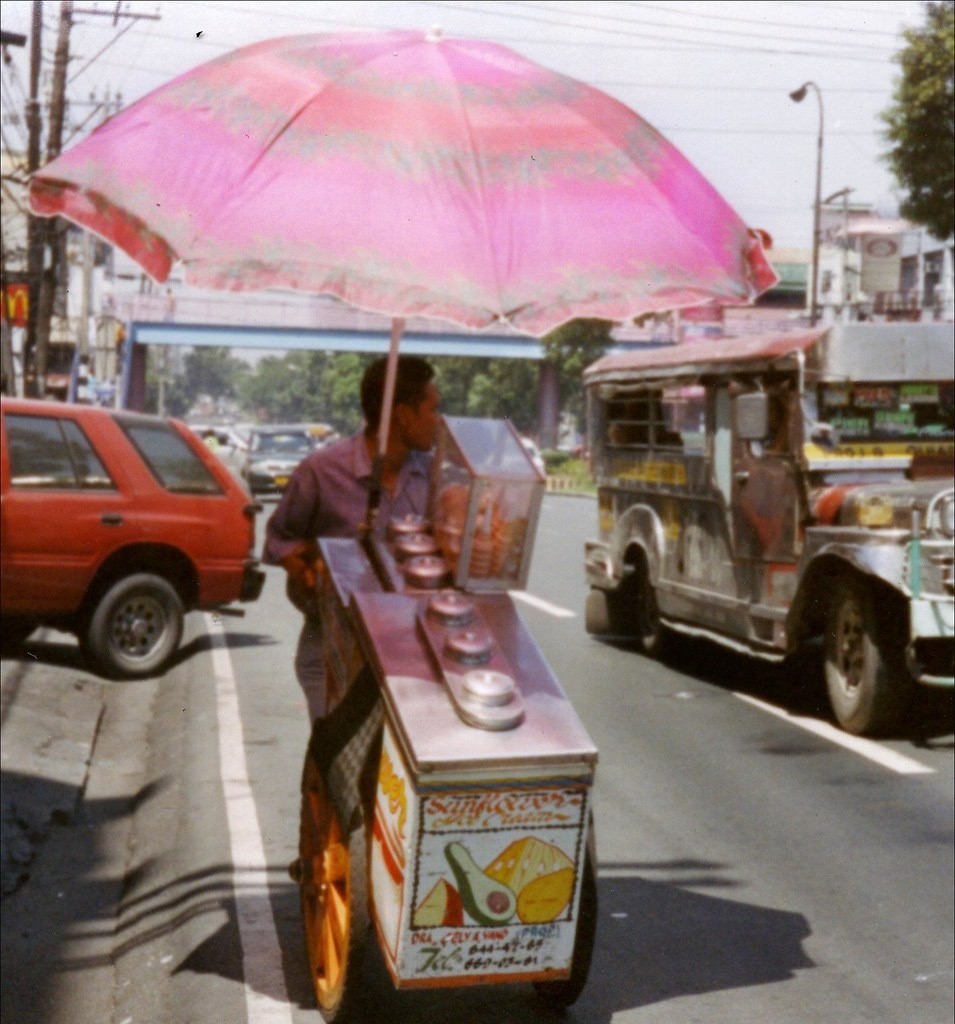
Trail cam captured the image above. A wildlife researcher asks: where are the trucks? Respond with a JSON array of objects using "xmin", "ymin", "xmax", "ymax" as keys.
[{"xmin": 568, "ymin": 315, "xmax": 955, "ymax": 744}]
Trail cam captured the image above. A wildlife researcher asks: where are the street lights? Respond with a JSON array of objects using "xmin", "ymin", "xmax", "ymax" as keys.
[{"xmin": 787, "ymin": 77, "xmax": 830, "ymax": 324}]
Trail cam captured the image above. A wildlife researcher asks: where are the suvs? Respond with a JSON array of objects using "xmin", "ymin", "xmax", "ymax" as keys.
[{"xmin": 0, "ymin": 392, "xmax": 273, "ymax": 686}]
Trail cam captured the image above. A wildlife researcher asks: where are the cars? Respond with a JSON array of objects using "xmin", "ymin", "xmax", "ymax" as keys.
[{"xmin": 180, "ymin": 420, "xmax": 343, "ymax": 501}]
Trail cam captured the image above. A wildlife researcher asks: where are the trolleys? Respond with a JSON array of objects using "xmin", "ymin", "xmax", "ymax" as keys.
[{"xmin": 298, "ymin": 530, "xmax": 606, "ymax": 1024}]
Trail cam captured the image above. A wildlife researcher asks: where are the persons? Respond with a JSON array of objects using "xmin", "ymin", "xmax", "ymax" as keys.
[
  {"xmin": 257, "ymin": 353, "xmax": 456, "ymax": 880},
  {"xmin": 200, "ymin": 428, "xmax": 235, "ymax": 468}
]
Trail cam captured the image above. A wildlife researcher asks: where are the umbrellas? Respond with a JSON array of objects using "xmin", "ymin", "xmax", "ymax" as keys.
[{"xmin": 22, "ymin": 26, "xmax": 786, "ymax": 533}]
